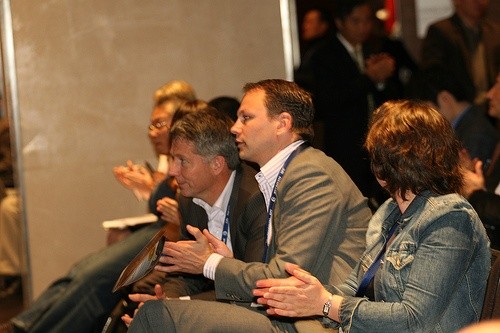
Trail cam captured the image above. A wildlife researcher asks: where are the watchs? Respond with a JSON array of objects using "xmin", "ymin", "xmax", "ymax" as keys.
[{"xmin": 323, "ymin": 294, "xmax": 335, "ymax": 317}]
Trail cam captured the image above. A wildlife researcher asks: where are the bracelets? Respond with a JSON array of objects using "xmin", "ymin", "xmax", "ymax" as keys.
[{"xmin": 253, "ymin": 99, "xmax": 492, "ymax": 333}]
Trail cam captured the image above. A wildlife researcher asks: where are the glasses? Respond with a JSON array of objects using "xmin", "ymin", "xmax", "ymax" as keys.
[{"xmin": 148, "ymin": 118, "xmax": 173, "ymax": 131}]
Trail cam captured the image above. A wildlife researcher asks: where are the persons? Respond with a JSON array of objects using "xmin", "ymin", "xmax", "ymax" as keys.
[{"xmin": 0, "ymin": 0, "xmax": 500, "ymax": 333}]
